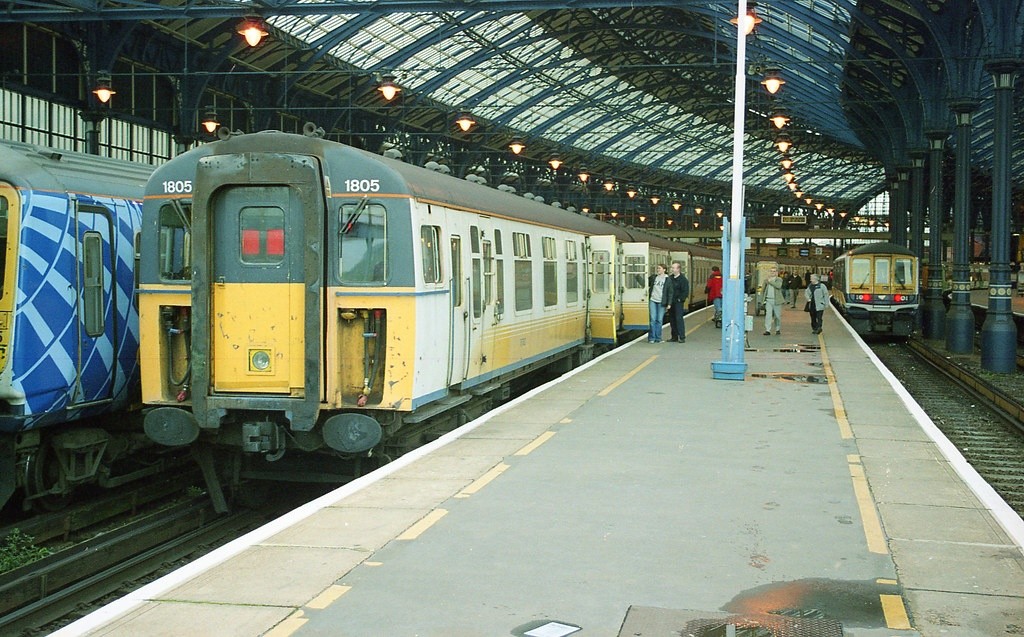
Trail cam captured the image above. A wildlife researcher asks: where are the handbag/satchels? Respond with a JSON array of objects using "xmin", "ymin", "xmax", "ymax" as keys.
[
  {"xmin": 761, "ymin": 300, "xmax": 765, "ymax": 309},
  {"xmin": 804, "ymin": 302, "xmax": 810, "ymax": 312}
]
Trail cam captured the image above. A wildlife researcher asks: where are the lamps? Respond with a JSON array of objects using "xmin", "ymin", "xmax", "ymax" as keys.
[
  {"xmin": 235, "ymin": 12, "xmax": 268, "ymax": 46},
  {"xmin": 509, "ymin": 132, "xmax": 526, "ymax": 153},
  {"xmin": 761, "ymin": 66, "xmax": 785, "ymax": 94},
  {"xmin": 91, "ymin": 73, "xmax": 116, "ymax": 103},
  {"xmin": 549, "ymin": 151, "xmax": 724, "ymax": 230},
  {"xmin": 730, "ymin": 0, "xmax": 762, "ymax": 35},
  {"xmin": 377, "ymin": 70, "xmax": 402, "ymax": 101},
  {"xmin": 201, "ymin": 110, "xmax": 221, "ymax": 133},
  {"xmin": 456, "ymin": 106, "xmax": 475, "ymax": 131},
  {"xmin": 770, "ymin": 105, "xmax": 790, "ymax": 128},
  {"xmin": 776, "ymin": 130, "xmax": 890, "ymax": 227}
]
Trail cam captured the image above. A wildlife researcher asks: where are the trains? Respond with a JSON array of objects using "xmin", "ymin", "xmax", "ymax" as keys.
[
  {"xmin": 0, "ymin": 137, "xmax": 192, "ymax": 512},
  {"xmin": 832, "ymin": 242, "xmax": 990, "ymax": 336},
  {"xmin": 131, "ymin": 121, "xmax": 831, "ymax": 513}
]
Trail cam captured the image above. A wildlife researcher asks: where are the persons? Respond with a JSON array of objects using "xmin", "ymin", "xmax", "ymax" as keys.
[
  {"xmin": 634, "ymin": 264, "xmax": 673, "ymax": 343},
  {"xmin": 823, "ymin": 269, "xmax": 833, "ymax": 288},
  {"xmin": 704, "ymin": 266, "xmax": 721, "ymax": 322},
  {"xmin": 779, "ymin": 269, "xmax": 802, "ymax": 308},
  {"xmin": 758, "ymin": 266, "xmax": 783, "ymax": 335},
  {"xmin": 805, "ymin": 270, "xmax": 812, "ymax": 289},
  {"xmin": 804, "ymin": 274, "xmax": 830, "ymax": 335},
  {"xmin": 668, "ymin": 262, "xmax": 689, "ymax": 342}
]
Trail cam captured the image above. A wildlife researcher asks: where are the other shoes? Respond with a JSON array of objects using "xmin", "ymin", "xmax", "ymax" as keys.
[
  {"xmin": 763, "ymin": 332, "xmax": 770, "ymax": 335},
  {"xmin": 787, "ymin": 301, "xmax": 790, "ymax": 304},
  {"xmin": 712, "ymin": 318, "xmax": 716, "ymax": 321},
  {"xmin": 649, "ymin": 340, "xmax": 655, "ymax": 344},
  {"xmin": 815, "ymin": 328, "xmax": 822, "ymax": 334},
  {"xmin": 679, "ymin": 339, "xmax": 685, "ymax": 343},
  {"xmin": 812, "ymin": 330, "xmax": 816, "ymax": 334},
  {"xmin": 667, "ymin": 339, "xmax": 678, "ymax": 342},
  {"xmin": 776, "ymin": 331, "xmax": 780, "ymax": 335},
  {"xmin": 791, "ymin": 305, "xmax": 795, "ymax": 308},
  {"xmin": 655, "ymin": 340, "xmax": 665, "ymax": 343}
]
